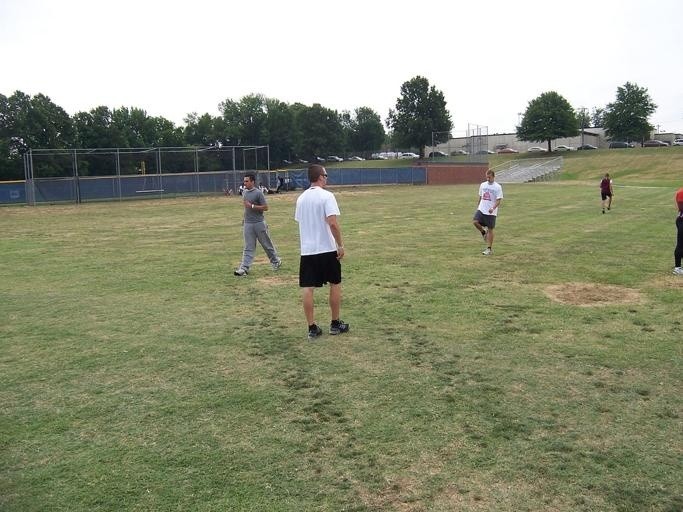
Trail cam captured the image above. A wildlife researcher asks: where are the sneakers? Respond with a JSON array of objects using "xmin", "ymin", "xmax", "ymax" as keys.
[
  {"xmin": 234, "ymin": 269, "xmax": 246, "ymax": 276},
  {"xmin": 482, "ymin": 248, "xmax": 493, "ymax": 255},
  {"xmin": 483, "ymin": 229, "xmax": 488, "ymax": 240},
  {"xmin": 308, "ymin": 325, "xmax": 322, "ymax": 340},
  {"xmin": 272, "ymin": 261, "xmax": 282, "ymax": 271},
  {"xmin": 329, "ymin": 320, "xmax": 349, "ymax": 335},
  {"xmin": 672, "ymin": 267, "xmax": 683, "ymax": 275}
]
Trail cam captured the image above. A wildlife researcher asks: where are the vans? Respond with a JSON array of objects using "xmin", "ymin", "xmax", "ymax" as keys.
[{"xmin": 607, "ymin": 141, "xmax": 635, "ymax": 148}]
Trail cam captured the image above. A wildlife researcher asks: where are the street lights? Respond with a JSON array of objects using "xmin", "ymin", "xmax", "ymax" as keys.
[{"xmin": 517, "ymin": 112, "xmax": 527, "ymax": 142}]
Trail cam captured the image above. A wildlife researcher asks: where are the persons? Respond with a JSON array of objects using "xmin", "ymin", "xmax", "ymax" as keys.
[
  {"xmin": 671, "ymin": 187, "xmax": 682, "ymax": 276},
  {"xmin": 599, "ymin": 172, "xmax": 615, "ymax": 215},
  {"xmin": 293, "ymin": 164, "xmax": 349, "ymax": 341},
  {"xmin": 233, "ymin": 174, "xmax": 282, "ymax": 277},
  {"xmin": 238, "ymin": 174, "xmax": 285, "ymax": 196},
  {"xmin": 472, "ymin": 169, "xmax": 503, "ymax": 255}
]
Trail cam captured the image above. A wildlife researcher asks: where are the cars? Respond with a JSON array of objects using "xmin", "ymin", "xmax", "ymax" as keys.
[
  {"xmin": 278, "ymin": 151, "xmax": 420, "ymax": 166},
  {"xmin": 640, "ymin": 139, "xmax": 669, "ymax": 147},
  {"xmin": 552, "ymin": 144, "xmax": 574, "ymax": 152},
  {"xmin": 576, "ymin": 144, "xmax": 597, "ymax": 150},
  {"xmin": 428, "ymin": 147, "xmax": 519, "ymax": 159},
  {"xmin": 527, "ymin": 147, "xmax": 548, "ymax": 152}
]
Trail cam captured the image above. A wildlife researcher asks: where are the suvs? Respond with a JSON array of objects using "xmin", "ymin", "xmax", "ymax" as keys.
[{"xmin": 672, "ymin": 139, "xmax": 683, "ymax": 146}]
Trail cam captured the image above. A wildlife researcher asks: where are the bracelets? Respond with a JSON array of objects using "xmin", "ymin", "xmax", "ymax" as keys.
[
  {"xmin": 336, "ymin": 244, "xmax": 344, "ymax": 250},
  {"xmin": 251, "ymin": 203, "xmax": 255, "ymax": 210},
  {"xmin": 490, "ymin": 207, "xmax": 495, "ymax": 211}
]
